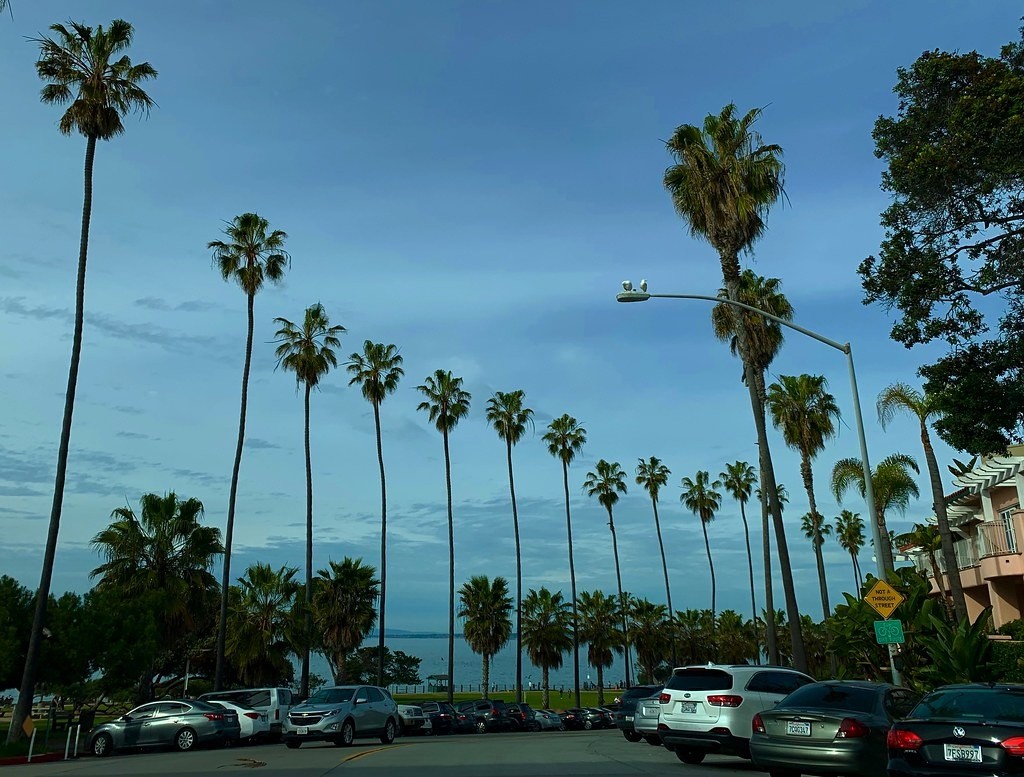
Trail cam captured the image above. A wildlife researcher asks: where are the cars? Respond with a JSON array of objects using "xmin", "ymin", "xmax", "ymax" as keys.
[
  {"xmin": 85, "ymin": 698, "xmax": 241, "ymax": 759},
  {"xmin": 887, "ymin": 683, "xmax": 1024, "ymax": 776},
  {"xmin": 205, "ymin": 700, "xmax": 263, "ymax": 743},
  {"xmin": 749, "ymin": 679, "xmax": 923, "ymax": 777}
]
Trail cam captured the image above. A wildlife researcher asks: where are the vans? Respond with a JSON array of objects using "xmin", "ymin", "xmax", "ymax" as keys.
[{"xmin": 196, "ymin": 689, "xmax": 292, "ymax": 738}]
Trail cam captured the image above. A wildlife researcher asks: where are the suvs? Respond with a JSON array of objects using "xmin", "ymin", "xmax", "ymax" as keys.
[
  {"xmin": 418, "ymin": 699, "xmax": 618, "ymax": 736},
  {"xmin": 617, "ymin": 683, "xmax": 664, "ymax": 743},
  {"xmin": 283, "ymin": 684, "xmax": 400, "ymax": 748},
  {"xmin": 657, "ymin": 662, "xmax": 817, "ymax": 767},
  {"xmin": 394, "ymin": 704, "xmax": 424, "ymax": 737}
]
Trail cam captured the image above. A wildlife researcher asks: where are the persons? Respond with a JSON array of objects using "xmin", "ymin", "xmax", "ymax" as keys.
[
  {"xmin": 1, "ymin": 694, "xmax": 13, "ymax": 717},
  {"xmin": 559, "ymin": 687, "xmax": 563, "ymax": 699},
  {"xmin": 614, "ymin": 696, "xmax": 621, "ymax": 703},
  {"xmin": 583, "ymin": 678, "xmax": 632, "ymax": 690},
  {"xmin": 528, "ymin": 680, "xmax": 532, "ymax": 691},
  {"xmin": 46, "ymin": 693, "xmax": 59, "ymax": 718},
  {"xmin": 491, "ymin": 681, "xmax": 495, "ymax": 691},
  {"xmin": 568, "ymin": 688, "xmax": 572, "ymax": 699}
]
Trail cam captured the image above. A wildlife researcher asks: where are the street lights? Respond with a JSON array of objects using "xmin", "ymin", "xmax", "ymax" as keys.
[{"xmin": 616, "ymin": 276, "xmax": 905, "ymax": 677}]
